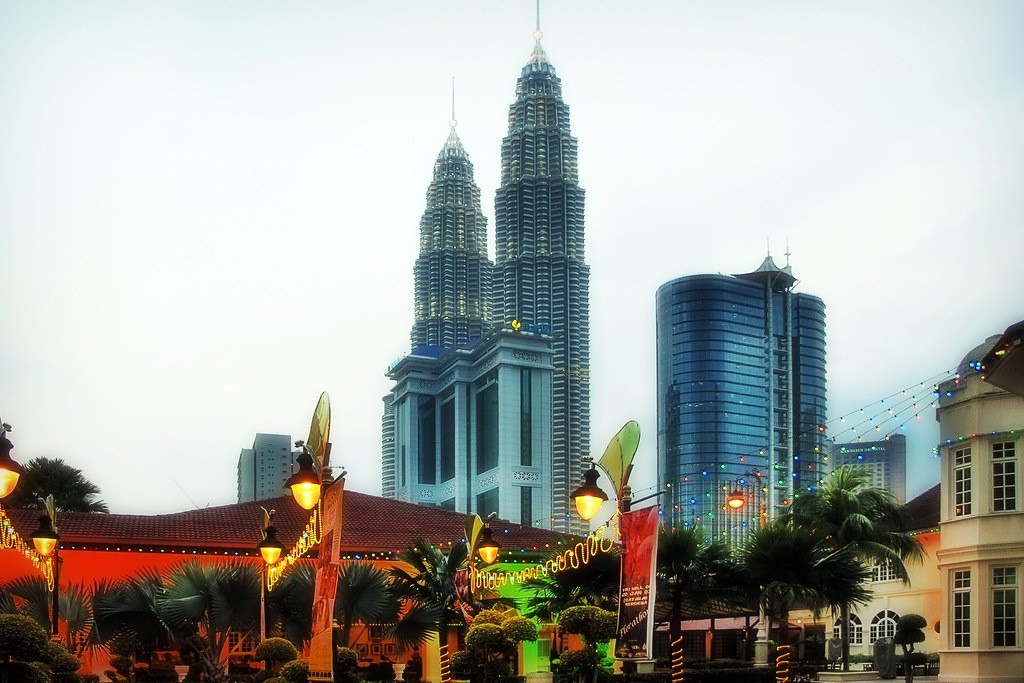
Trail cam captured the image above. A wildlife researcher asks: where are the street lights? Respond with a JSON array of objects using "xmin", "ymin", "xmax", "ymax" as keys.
[
  {"xmin": 728, "ymin": 472, "xmax": 774, "ymax": 669},
  {"xmin": 464, "ymin": 512, "xmax": 499, "ymax": 609},
  {"xmin": 259, "ymin": 509, "xmax": 283, "ymax": 640},
  {"xmin": 570, "ymin": 464, "xmax": 636, "ymax": 676},
  {"xmin": 282, "ymin": 442, "xmax": 338, "ymax": 683},
  {"xmin": 29, "ymin": 493, "xmax": 60, "ymax": 644}
]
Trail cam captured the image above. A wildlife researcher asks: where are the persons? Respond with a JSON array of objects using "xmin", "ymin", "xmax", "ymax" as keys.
[
  {"xmin": 360, "ymin": 654, "xmax": 391, "ymax": 663},
  {"xmin": 564, "ymin": 646, "xmax": 569, "ymax": 653},
  {"xmin": 412, "ymin": 646, "xmax": 422, "ymax": 660}
]
